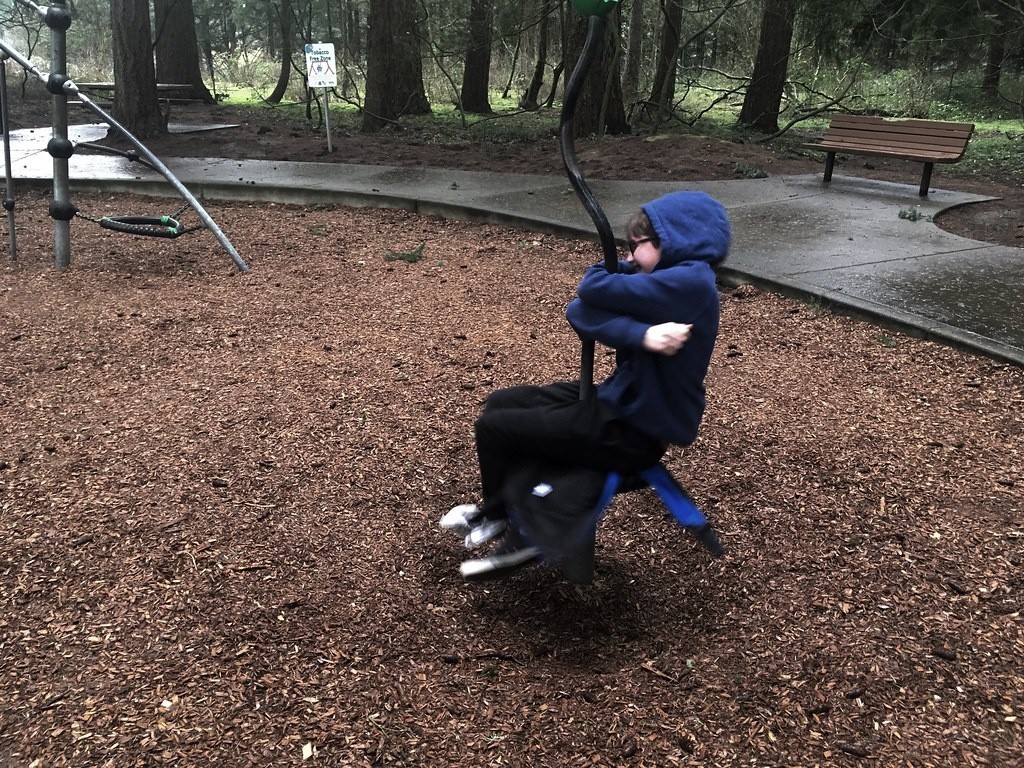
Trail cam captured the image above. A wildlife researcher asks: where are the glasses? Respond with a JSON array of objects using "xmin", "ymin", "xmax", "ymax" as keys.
[{"xmin": 627, "ymin": 235, "xmax": 650, "ymax": 256}]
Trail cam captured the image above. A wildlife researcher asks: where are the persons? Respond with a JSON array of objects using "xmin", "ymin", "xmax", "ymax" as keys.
[{"xmin": 439, "ymin": 192, "xmax": 732, "ymax": 579}]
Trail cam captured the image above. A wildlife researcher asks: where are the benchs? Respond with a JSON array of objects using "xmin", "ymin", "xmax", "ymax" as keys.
[{"xmin": 801, "ymin": 113, "xmax": 975, "ymax": 197}]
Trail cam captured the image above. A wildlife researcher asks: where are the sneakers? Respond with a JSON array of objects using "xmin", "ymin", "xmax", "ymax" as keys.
[{"xmin": 437, "ymin": 499, "xmax": 545, "ymax": 583}]
